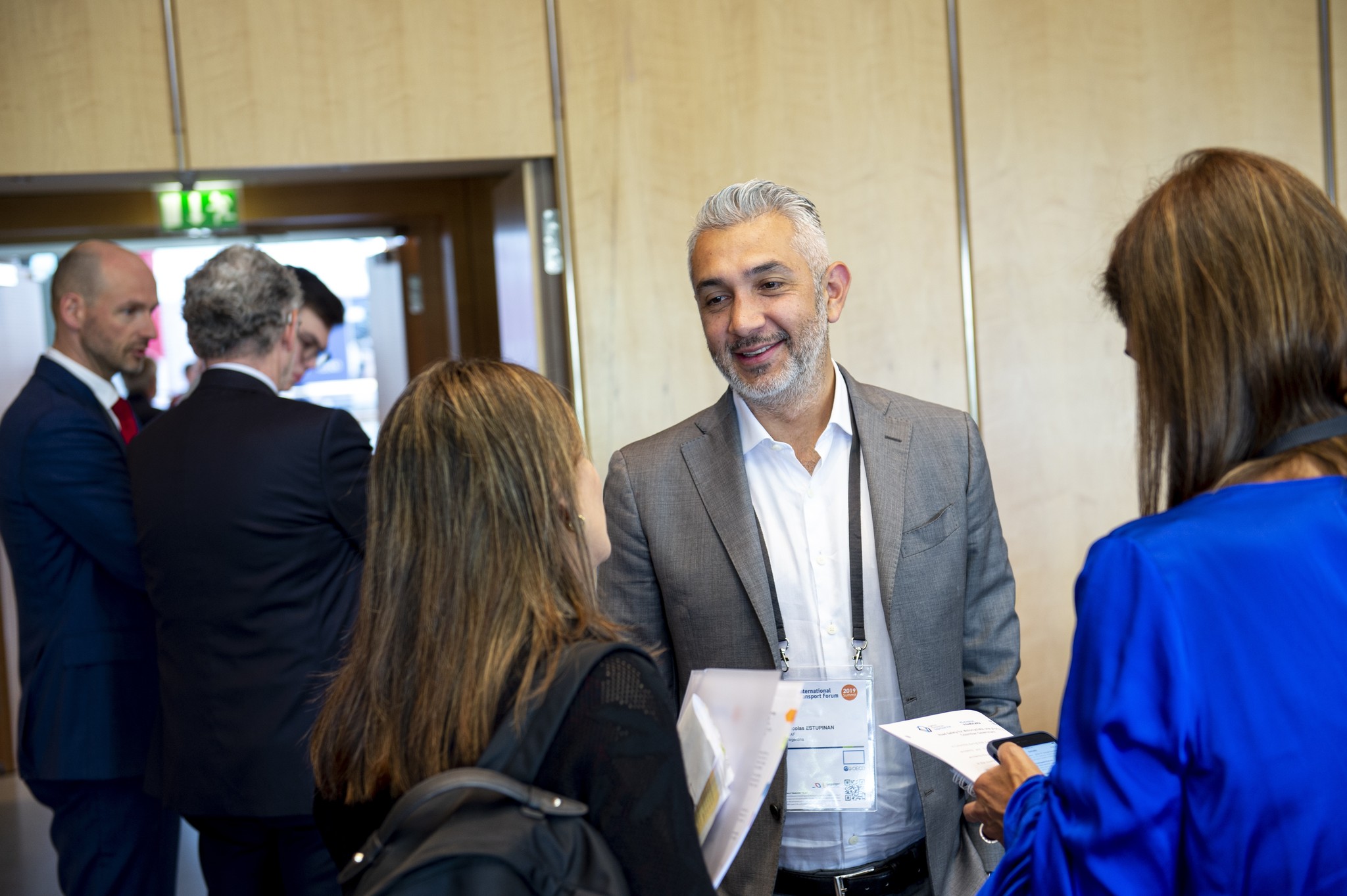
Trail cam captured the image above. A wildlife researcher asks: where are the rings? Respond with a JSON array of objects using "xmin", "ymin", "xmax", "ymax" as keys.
[{"xmin": 979, "ymin": 823, "xmax": 998, "ymax": 845}]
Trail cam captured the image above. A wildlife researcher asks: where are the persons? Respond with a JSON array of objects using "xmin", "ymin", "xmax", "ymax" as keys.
[
  {"xmin": 272, "ymin": 266, "xmax": 344, "ymax": 394},
  {"xmin": 309, "ymin": 359, "xmax": 718, "ymax": 896},
  {"xmin": 116, "ymin": 246, "xmax": 372, "ymax": 896},
  {"xmin": 598, "ymin": 180, "xmax": 1020, "ymax": 896},
  {"xmin": 109, "ymin": 358, "xmax": 199, "ymax": 444},
  {"xmin": 962, "ymin": 148, "xmax": 1347, "ymax": 896},
  {"xmin": 0, "ymin": 238, "xmax": 170, "ymax": 896}
]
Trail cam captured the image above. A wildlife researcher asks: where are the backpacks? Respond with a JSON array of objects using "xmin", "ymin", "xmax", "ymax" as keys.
[{"xmin": 333, "ymin": 634, "xmax": 658, "ymax": 896}]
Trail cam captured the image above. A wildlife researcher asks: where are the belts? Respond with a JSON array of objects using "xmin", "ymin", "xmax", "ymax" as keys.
[{"xmin": 772, "ymin": 841, "xmax": 928, "ymax": 896}]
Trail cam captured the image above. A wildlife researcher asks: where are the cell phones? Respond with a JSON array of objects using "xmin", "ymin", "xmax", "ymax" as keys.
[{"xmin": 987, "ymin": 731, "xmax": 1057, "ymax": 777}]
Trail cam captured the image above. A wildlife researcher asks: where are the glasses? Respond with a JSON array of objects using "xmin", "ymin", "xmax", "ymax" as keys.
[{"xmin": 296, "ymin": 331, "xmax": 330, "ymax": 370}]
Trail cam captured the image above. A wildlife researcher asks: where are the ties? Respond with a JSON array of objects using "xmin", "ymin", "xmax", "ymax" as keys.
[{"xmin": 110, "ymin": 398, "xmax": 141, "ymax": 444}]
